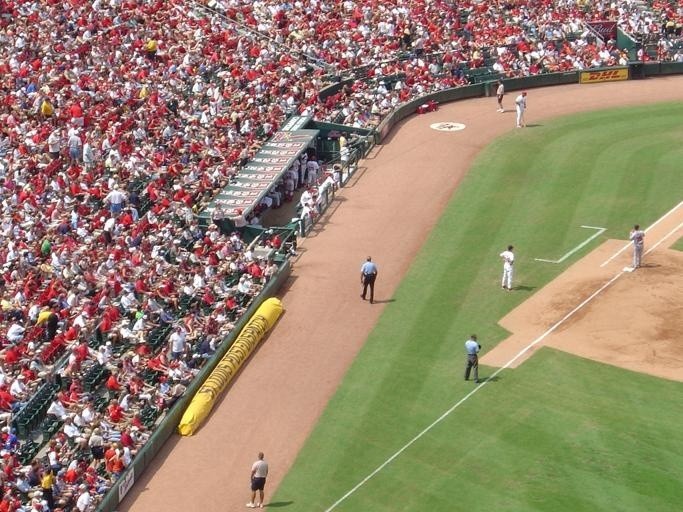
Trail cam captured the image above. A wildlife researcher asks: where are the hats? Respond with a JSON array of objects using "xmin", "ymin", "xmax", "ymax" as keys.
[{"xmin": 508, "ymin": 245, "xmax": 514, "ymax": 250}]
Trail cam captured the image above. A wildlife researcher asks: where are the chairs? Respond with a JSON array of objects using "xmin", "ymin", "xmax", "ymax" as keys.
[
  {"xmin": 8, "ymin": 319, "xmax": 169, "ymax": 470},
  {"xmin": 368, "ymin": 59, "xmax": 520, "ymax": 94}
]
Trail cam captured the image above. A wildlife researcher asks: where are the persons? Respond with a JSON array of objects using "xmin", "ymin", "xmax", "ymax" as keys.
[
  {"xmin": 463, "ymin": 334, "xmax": 481, "ymax": 384},
  {"xmin": 358, "ymin": 255, "xmax": 378, "ymax": 306},
  {"xmin": 629, "ymin": 225, "xmax": 645, "ymax": 270},
  {"xmin": 3, "ymin": 0, "xmax": 682, "ymax": 221},
  {"xmin": 500, "ymin": 245, "xmax": 514, "ymax": 291},
  {"xmin": 0, "ymin": 191, "xmax": 293, "ymax": 512}
]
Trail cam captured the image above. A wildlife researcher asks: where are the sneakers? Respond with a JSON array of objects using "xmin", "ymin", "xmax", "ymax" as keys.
[
  {"xmin": 496, "ymin": 109, "xmax": 504, "ymax": 113},
  {"xmin": 246, "ymin": 502, "xmax": 264, "ymax": 509}
]
